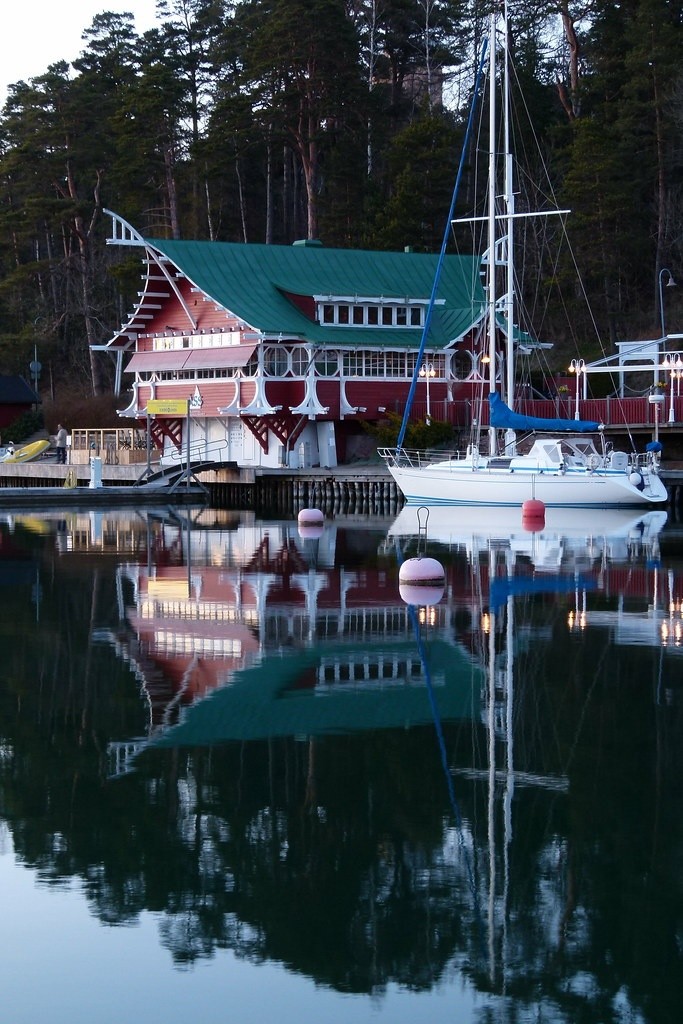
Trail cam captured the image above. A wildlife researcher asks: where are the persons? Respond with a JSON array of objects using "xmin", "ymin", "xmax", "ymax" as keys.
[
  {"xmin": 54, "ymin": 424, "xmax": 67, "ymax": 464},
  {"xmin": 1, "ymin": 441, "xmax": 14, "ymax": 460}
]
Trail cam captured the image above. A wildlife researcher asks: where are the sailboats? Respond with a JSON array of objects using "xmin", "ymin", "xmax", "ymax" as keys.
[
  {"xmin": 387, "ymin": 501, "xmax": 672, "ymax": 1015},
  {"xmin": 378, "ymin": 1, "xmax": 674, "ymax": 506}
]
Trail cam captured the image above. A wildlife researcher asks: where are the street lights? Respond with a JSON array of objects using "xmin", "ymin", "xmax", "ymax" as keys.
[
  {"xmin": 419, "ymin": 362, "xmax": 436, "ymax": 427},
  {"xmin": 567, "ymin": 358, "xmax": 588, "ymax": 421},
  {"xmin": 661, "ymin": 353, "xmax": 683, "ymax": 426},
  {"xmin": 656, "ymin": 267, "xmax": 677, "ymax": 390}
]
